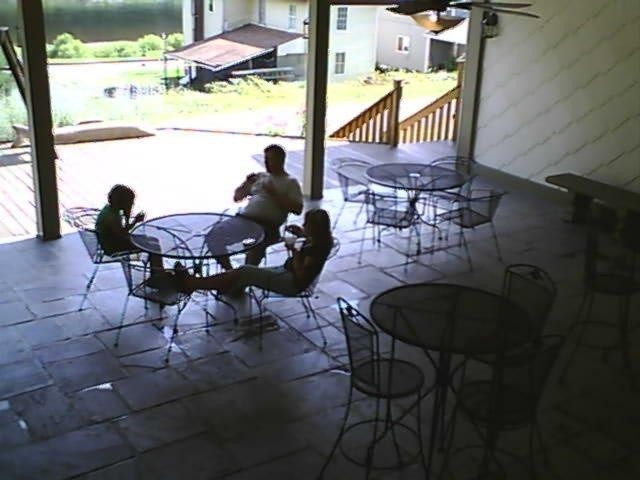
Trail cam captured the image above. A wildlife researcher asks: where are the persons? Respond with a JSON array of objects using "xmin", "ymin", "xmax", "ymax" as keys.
[
  {"xmin": 94, "ymin": 184, "xmax": 163, "ymax": 277},
  {"xmin": 163, "ymin": 207, "xmax": 334, "ymax": 297},
  {"xmin": 202, "ymin": 144, "xmax": 304, "ymax": 269}
]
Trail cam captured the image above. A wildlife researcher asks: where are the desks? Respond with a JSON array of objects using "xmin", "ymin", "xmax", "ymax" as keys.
[
  {"xmin": 128, "ymin": 211, "xmax": 268, "ymax": 337},
  {"xmin": 366, "ymin": 160, "xmax": 466, "ymax": 257}
]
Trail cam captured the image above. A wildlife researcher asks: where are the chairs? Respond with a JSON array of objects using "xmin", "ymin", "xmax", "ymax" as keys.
[
  {"xmin": 329, "ymin": 163, "xmax": 399, "ymax": 233},
  {"xmin": 427, "ymin": 186, "xmax": 511, "ymax": 273},
  {"xmin": 356, "ymin": 189, "xmax": 424, "ymax": 276},
  {"xmin": 253, "ymin": 236, "xmax": 331, "ymax": 349},
  {"xmin": 413, "ymin": 154, "xmax": 479, "ymax": 243},
  {"xmin": 115, "ymin": 256, "xmax": 220, "ymax": 372},
  {"xmin": 62, "ymin": 199, "xmax": 143, "ymax": 317},
  {"xmin": 209, "ymin": 204, "xmax": 303, "ymax": 302}
]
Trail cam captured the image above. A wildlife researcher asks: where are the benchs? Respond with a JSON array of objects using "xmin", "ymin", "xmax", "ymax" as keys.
[{"xmin": 544, "ymin": 172, "xmax": 640, "ymax": 236}]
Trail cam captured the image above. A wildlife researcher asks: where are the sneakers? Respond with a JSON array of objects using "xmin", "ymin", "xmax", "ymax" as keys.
[
  {"xmin": 174, "ymin": 261, "xmax": 191, "ymax": 294},
  {"xmin": 150, "ymin": 270, "xmax": 172, "ymax": 279}
]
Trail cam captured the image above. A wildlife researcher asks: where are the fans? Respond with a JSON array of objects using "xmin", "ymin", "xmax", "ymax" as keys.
[{"xmin": 327, "ymin": 0, "xmax": 544, "ymax": 18}]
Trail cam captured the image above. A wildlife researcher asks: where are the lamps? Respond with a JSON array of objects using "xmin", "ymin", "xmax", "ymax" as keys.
[
  {"xmin": 411, "ymin": 9, "xmax": 465, "ymax": 34},
  {"xmin": 480, "ymin": 11, "xmax": 499, "ymax": 41}
]
